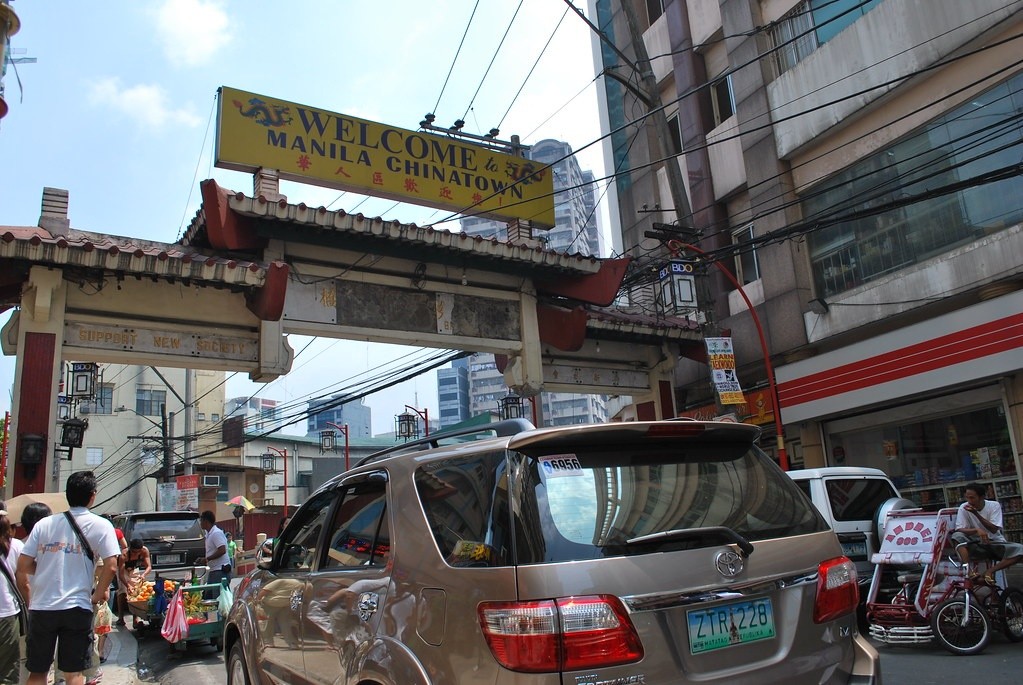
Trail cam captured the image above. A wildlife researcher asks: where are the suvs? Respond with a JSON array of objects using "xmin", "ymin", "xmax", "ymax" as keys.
[
  {"xmin": 786, "ymin": 467, "xmax": 926, "ymax": 603},
  {"xmin": 108, "ymin": 509, "xmax": 211, "ymax": 610},
  {"xmin": 223, "ymin": 416, "xmax": 882, "ymax": 685}
]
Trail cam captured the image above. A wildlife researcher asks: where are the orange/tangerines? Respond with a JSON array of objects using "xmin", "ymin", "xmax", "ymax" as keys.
[{"xmin": 137, "ymin": 580, "xmax": 175, "ymax": 600}]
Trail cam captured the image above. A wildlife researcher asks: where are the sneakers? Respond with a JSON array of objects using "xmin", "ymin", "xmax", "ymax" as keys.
[
  {"xmin": 290, "ymin": 644, "xmax": 300, "ymax": 650},
  {"xmin": 264, "ymin": 643, "xmax": 275, "ymax": 647}
]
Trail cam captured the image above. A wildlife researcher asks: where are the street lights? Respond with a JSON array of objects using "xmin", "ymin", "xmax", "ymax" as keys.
[
  {"xmin": 114, "ymin": 405, "xmax": 170, "ymax": 484},
  {"xmin": 658, "ymin": 240, "xmax": 789, "ymax": 472},
  {"xmin": 261, "ymin": 446, "xmax": 287, "ymax": 517},
  {"xmin": 394, "ymin": 407, "xmax": 428, "ymax": 444},
  {"xmin": 318, "ymin": 422, "xmax": 349, "ymax": 473}
]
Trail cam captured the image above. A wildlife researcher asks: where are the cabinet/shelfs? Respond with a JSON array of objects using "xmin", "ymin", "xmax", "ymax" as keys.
[{"xmin": 898, "ymin": 474, "xmax": 1023, "ymax": 544}]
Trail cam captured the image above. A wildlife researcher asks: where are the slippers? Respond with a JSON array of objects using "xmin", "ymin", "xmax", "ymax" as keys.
[{"xmin": 969, "ymin": 572, "xmax": 993, "ymax": 587}]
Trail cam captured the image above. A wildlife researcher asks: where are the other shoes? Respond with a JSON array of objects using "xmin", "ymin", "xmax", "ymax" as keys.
[
  {"xmin": 133, "ymin": 623, "xmax": 137, "ymax": 629},
  {"xmin": 115, "ymin": 618, "xmax": 126, "ymax": 626}
]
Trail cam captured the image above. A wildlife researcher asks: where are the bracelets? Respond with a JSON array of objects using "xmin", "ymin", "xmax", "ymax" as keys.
[{"xmin": 205, "ymin": 558, "xmax": 209, "ymax": 565}]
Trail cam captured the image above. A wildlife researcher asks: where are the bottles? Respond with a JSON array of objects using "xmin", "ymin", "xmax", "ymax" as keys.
[{"xmin": 945, "ymin": 483, "xmax": 1023, "ymax": 544}]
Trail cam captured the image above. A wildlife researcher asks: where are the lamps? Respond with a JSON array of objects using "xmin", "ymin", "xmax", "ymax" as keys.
[
  {"xmin": 55, "ymin": 417, "xmax": 89, "ymax": 461},
  {"xmin": 19, "ymin": 432, "xmax": 47, "ymax": 488},
  {"xmin": 808, "ymin": 298, "xmax": 828, "ymax": 314}
]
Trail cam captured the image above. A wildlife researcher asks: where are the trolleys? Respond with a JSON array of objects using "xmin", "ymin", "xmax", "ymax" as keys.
[{"xmin": 128, "ymin": 567, "xmax": 229, "ymax": 652}]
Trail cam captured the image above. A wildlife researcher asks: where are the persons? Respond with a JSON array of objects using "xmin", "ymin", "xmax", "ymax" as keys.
[
  {"xmin": 949, "ymin": 482, "xmax": 1023, "ymax": 583},
  {"xmin": 16, "ymin": 471, "xmax": 120, "ymax": 685},
  {"xmin": 277, "ymin": 517, "xmax": 291, "ymax": 537},
  {"xmin": 194, "ymin": 511, "xmax": 233, "ymax": 597},
  {"xmin": 225, "ymin": 532, "xmax": 237, "ymax": 566},
  {"xmin": 242, "ymin": 539, "xmax": 311, "ymax": 650},
  {"xmin": 0, "ymin": 501, "xmax": 152, "ymax": 685},
  {"xmin": 321, "ymin": 575, "xmax": 414, "ymax": 676}
]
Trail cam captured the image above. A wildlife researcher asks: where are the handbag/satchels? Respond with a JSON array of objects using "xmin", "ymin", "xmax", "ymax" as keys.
[
  {"xmin": 217, "ymin": 583, "xmax": 234, "ymax": 618},
  {"xmin": 18, "ymin": 600, "xmax": 31, "ymax": 636},
  {"xmin": 290, "ymin": 588, "xmax": 303, "ymax": 613},
  {"xmin": 94, "ymin": 601, "xmax": 112, "ymax": 635},
  {"xmin": 161, "ymin": 586, "xmax": 189, "ymax": 644}
]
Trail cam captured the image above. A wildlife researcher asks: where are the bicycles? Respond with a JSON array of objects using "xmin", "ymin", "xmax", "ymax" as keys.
[{"xmin": 931, "ymin": 539, "xmax": 1023, "ymax": 655}]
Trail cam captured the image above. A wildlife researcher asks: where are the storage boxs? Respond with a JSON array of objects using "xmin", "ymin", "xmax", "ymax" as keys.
[{"xmin": 970, "ymin": 446, "xmax": 1001, "ymax": 479}]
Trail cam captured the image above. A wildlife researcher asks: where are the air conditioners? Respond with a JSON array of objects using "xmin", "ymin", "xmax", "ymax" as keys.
[{"xmin": 203, "ymin": 475, "xmax": 220, "ymax": 487}]
